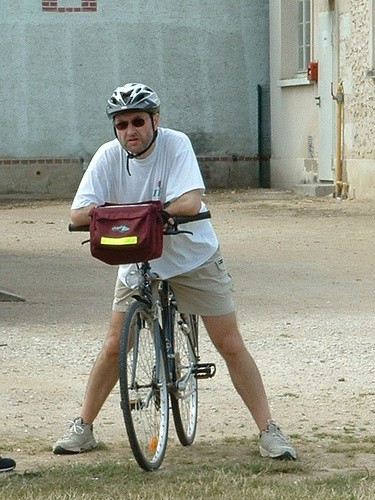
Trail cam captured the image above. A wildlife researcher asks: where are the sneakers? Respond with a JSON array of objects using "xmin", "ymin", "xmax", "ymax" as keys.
[
  {"xmin": 53, "ymin": 417, "xmax": 98, "ymax": 454},
  {"xmin": 259, "ymin": 423, "xmax": 297, "ymax": 460}
]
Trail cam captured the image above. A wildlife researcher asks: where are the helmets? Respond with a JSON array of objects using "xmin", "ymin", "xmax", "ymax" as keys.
[{"xmin": 106, "ymin": 82, "xmax": 161, "ymax": 120}]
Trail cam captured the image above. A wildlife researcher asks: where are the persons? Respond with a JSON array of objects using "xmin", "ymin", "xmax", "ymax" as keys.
[
  {"xmin": 51, "ymin": 83, "xmax": 298, "ymax": 462},
  {"xmin": 0, "ymin": 456, "xmax": 16, "ymax": 472}
]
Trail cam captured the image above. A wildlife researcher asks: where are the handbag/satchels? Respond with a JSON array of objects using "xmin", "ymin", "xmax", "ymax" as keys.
[{"xmin": 87, "ymin": 200, "xmax": 164, "ymax": 265}]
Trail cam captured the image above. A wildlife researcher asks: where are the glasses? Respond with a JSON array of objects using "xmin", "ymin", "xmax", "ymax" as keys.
[{"xmin": 114, "ymin": 117, "xmax": 151, "ymax": 130}]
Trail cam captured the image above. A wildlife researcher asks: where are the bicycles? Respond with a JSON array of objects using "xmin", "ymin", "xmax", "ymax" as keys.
[{"xmin": 67, "ymin": 202, "xmax": 213, "ymax": 472}]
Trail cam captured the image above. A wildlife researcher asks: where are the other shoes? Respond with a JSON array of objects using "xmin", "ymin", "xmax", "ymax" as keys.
[{"xmin": 0, "ymin": 456, "xmax": 16, "ymax": 472}]
